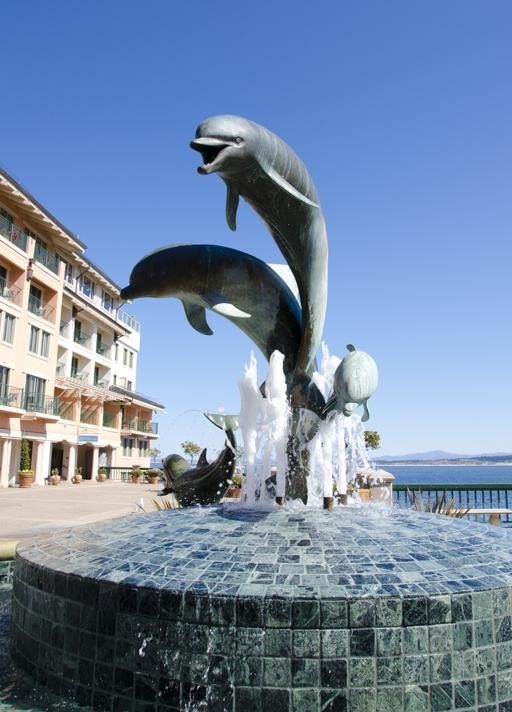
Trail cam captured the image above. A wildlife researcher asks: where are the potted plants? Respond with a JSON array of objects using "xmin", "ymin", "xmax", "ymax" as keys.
[
  {"xmin": 98, "ymin": 467, "xmax": 106, "ymax": 481},
  {"xmin": 17, "ymin": 440, "xmax": 33, "ymax": 487},
  {"xmin": 130, "ymin": 469, "xmax": 142, "ymax": 484},
  {"xmin": 145, "ymin": 469, "xmax": 161, "ymax": 484},
  {"xmin": 73, "ymin": 466, "xmax": 83, "ymax": 483},
  {"xmin": 227, "ymin": 476, "xmax": 241, "ymax": 498},
  {"xmin": 49, "ymin": 467, "xmax": 61, "ymax": 485}
]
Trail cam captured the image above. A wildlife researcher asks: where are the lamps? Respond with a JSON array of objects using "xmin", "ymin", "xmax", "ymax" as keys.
[{"xmin": 26, "ymin": 258, "xmax": 35, "ymax": 281}]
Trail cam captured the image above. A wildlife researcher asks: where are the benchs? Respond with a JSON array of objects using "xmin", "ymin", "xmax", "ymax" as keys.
[{"xmin": 437, "ymin": 506, "xmax": 512, "ymax": 526}]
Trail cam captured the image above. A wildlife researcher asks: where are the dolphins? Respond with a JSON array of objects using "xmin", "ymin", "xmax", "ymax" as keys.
[
  {"xmin": 121, "ymin": 243, "xmax": 304, "ymax": 388},
  {"xmin": 156, "ymin": 409, "xmax": 244, "ymax": 505},
  {"xmin": 320, "ymin": 343, "xmax": 379, "ymax": 422},
  {"xmin": 191, "ymin": 115, "xmax": 329, "ymax": 395}
]
